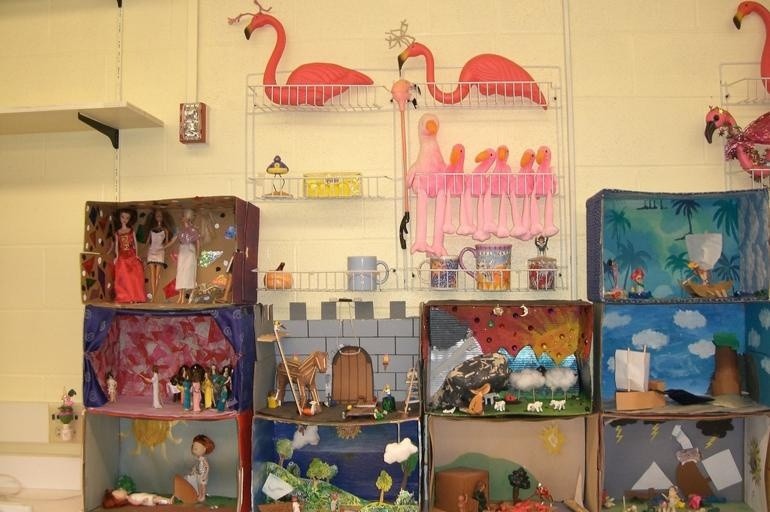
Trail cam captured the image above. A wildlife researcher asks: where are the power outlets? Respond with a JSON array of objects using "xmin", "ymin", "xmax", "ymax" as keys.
[{"xmin": 47, "ymin": 403, "xmax": 84, "ymax": 444}]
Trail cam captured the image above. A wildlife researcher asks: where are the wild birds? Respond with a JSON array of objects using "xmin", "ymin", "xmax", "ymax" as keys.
[
  {"xmin": 701, "ymin": 106, "xmax": 770, "ymax": 178},
  {"xmin": 243, "ymin": 12, "xmax": 375, "ymax": 107},
  {"xmin": 731, "ymin": 0, "xmax": 770, "ymax": 94},
  {"xmin": 396, "ymin": 41, "xmax": 548, "ymax": 106}
]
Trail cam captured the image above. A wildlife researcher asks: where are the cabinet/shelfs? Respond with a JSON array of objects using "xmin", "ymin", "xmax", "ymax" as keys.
[{"xmin": 244, "ymin": 68, "xmax": 570, "ymax": 293}]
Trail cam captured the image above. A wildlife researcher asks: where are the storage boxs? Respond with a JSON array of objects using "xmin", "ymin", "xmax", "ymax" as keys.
[
  {"xmin": 588, "ymin": 408, "xmax": 770, "ymax": 511},
  {"xmin": 417, "ymin": 296, "xmax": 597, "ymax": 418},
  {"xmin": 246, "ymin": 400, "xmax": 419, "ymax": 512},
  {"xmin": 77, "ymin": 189, "xmax": 256, "ymax": 309},
  {"xmin": 76, "ymin": 406, "xmax": 251, "ymax": 512},
  {"xmin": 418, "ymin": 411, "xmax": 599, "ymax": 512},
  {"xmin": 585, "ymin": 185, "xmax": 769, "ymax": 302},
  {"xmin": 583, "ymin": 299, "xmax": 770, "ymax": 416},
  {"xmin": 78, "ymin": 304, "xmax": 254, "ymax": 420}
]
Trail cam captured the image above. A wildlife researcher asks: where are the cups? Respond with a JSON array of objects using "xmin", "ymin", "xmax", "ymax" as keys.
[
  {"xmin": 458, "ymin": 244, "xmax": 512, "ymax": 293},
  {"xmin": 346, "ymin": 255, "xmax": 389, "ymax": 291},
  {"xmin": 416, "ymin": 255, "xmax": 460, "ymax": 289}
]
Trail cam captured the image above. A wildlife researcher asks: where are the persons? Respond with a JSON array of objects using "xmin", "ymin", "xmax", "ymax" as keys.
[
  {"xmin": 471, "ymin": 479, "xmax": 487, "ymax": 512},
  {"xmin": 106, "ymin": 487, "xmax": 175, "ymax": 508},
  {"xmin": 106, "ymin": 371, "xmax": 118, "ymax": 403},
  {"xmin": 606, "ymin": 259, "xmax": 647, "ymax": 296},
  {"xmin": 139, "ymin": 364, "xmax": 234, "ymax": 414},
  {"xmin": 191, "ymin": 434, "xmax": 216, "ymax": 503},
  {"xmin": 110, "ymin": 207, "xmax": 202, "ymax": 304},
  {"xmin": 659, "ymin": 486, "xmax": 702, "ymax": 512}
]
